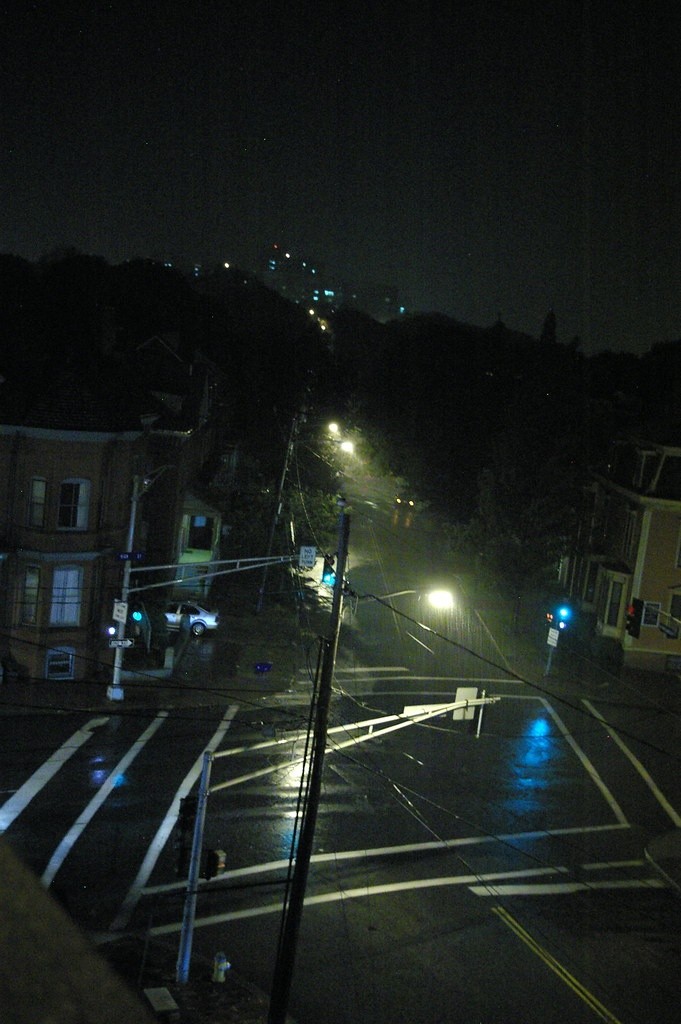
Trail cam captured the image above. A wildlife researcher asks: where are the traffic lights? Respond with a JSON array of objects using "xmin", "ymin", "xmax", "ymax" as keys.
[
  {"xmin": 554, "ymin": 600, "xmax": 573, "ymax": 632},
  {"xmin": 131, "ymin": 607, "xmax": 141, "ymax": 626},
  {"xmin": 393, "ymin": 496, "xmax": 416, "ymax": 508},
  {"xmin": 625, "ymin": 598, "xmax": 647, "ymax": 639},
  {"xmin": 323, "ymin": 555, "xmax": 335, "ymax": 583}
]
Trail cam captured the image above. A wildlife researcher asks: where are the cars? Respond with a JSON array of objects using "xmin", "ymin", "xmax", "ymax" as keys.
[{"xmin": 163, "ymin": 601, "xmax": 220, "ymax": 637}]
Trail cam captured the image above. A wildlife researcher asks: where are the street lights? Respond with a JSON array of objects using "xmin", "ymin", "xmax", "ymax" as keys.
[{"xmin": 263, "ymin": 571, "xmax": 470, "ymax": 1024}]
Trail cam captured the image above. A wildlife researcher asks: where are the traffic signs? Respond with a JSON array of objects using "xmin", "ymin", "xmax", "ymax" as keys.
[{"xmin": 109, "ymin": 637, "xmax": 134, "ymax": 649}]
